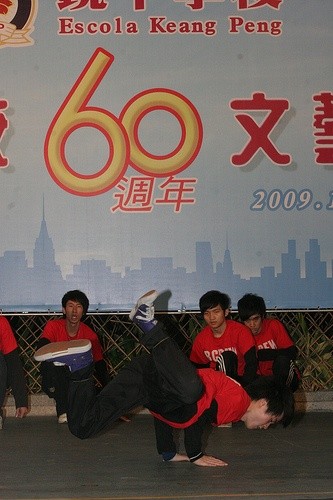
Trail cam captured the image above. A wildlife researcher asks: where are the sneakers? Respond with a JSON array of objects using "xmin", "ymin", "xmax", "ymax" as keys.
[
  {"xmin": 58, "ymin": 412, "xmax": 68, "ymax": 424},
  {"xmin": 129, "ymin": 290, "xmax": 158, "ymax": 332},
  {"xmin": 33, "ymin": 339, "xmax": 93, "ymax": 372}
]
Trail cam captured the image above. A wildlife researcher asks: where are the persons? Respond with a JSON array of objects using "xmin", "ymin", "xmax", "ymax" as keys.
[
  {"xmin": 33, "ymin": 289, "xmax": 294, "ymax": 467},
  {"xmin": 38, "ymin": 290, "xmax": 130, "ymax": 423},
  {"xmin": 188, "ymin": 290, "xmax": 300, "ymax": 427},
  {"xmin": 0, "ymin": 316, "xmax": 28, "ymax": 430}
]
